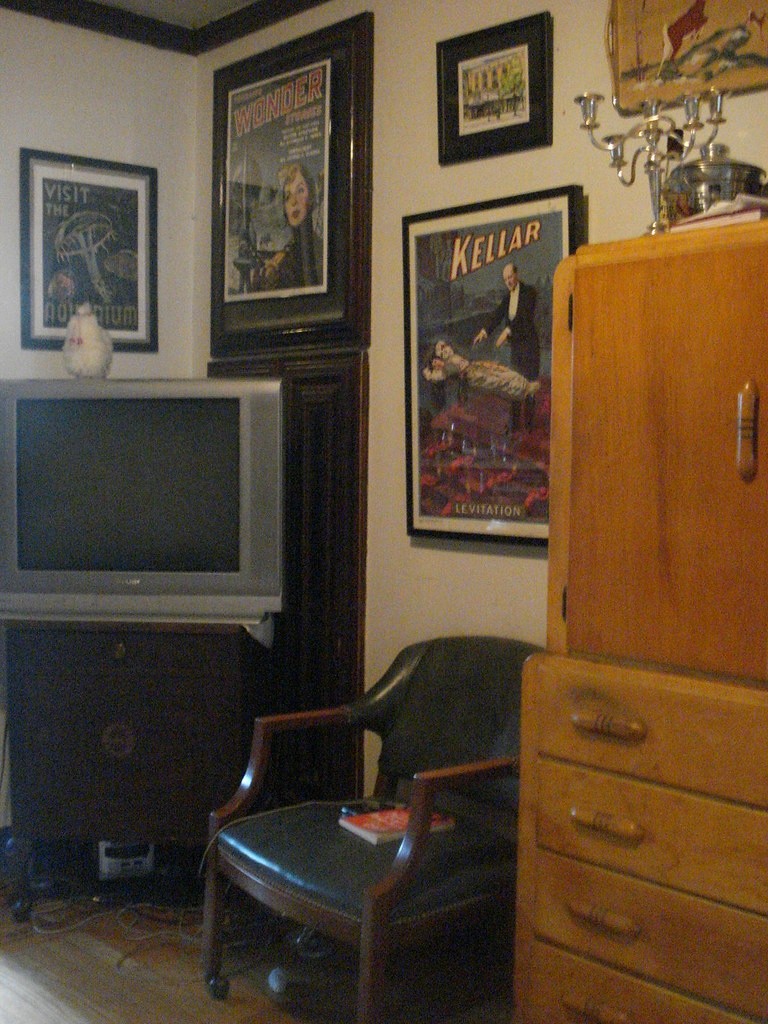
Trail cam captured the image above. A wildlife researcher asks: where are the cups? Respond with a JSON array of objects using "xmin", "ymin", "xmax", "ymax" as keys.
[{"xmin": 671, "ymin": 141, "xmax": 764, "ymax": 214}]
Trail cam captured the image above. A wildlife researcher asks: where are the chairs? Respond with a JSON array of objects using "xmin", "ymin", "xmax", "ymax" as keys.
[{"xmin": 200, "ymin": 637, "xmax": 543, "ymax": 1023}]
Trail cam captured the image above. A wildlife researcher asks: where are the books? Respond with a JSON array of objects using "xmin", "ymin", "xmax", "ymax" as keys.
[
  {"xmin": 339, "ymin": 803, "xmax": 456, "ymax": 846},
  {"xmin": 665, "ymin": 207, "xmax": 768, "ymax": 239}
]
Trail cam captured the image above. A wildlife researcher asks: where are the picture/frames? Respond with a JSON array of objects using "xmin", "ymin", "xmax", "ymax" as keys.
[
  {"xmin": 210, "ymin": 10, "xmax": 376, "ymax": 358},
  {"xmin": 401, "ymin": 182, "xmax": 586, "ymax": 545},
  {"xmin": 17, "ymin": 145, "xmax": 161, "ymax": 354},
  {"xmin": 436, "ymin": 11, "xmax": 555, "ymax": 166}
]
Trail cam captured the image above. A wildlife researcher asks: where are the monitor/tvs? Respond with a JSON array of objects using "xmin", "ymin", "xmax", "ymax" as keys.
[{"xmin": 0, "ymin": 376, "xmax": 288, "ymax": 624}]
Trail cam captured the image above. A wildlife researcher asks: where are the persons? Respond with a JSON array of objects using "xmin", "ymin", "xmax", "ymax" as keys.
[
  {"xmin": 423, "ymin": 341, "xmax": 539, "ymax": 411},
  {"xmin": 473, "ymin": 261, "xmax": 540, "ymax": 437}
]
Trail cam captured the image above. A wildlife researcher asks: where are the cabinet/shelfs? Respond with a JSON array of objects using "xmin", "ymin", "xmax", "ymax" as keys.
[
  {"xmin": 514, "ymin": 215, "xmax": 768, "ymax": 1022},
  {"xmin": 4, "ymin": 619, "xmax": 242, "ymax": 919}
]
